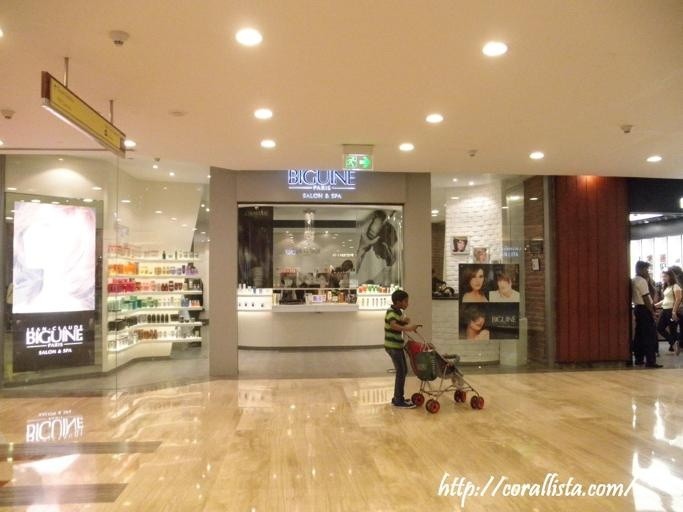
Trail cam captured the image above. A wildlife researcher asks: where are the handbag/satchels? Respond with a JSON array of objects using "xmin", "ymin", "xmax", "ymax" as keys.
[{"xmin": 672, "ymin": 285, "xmax": 683, "ymax": 317}]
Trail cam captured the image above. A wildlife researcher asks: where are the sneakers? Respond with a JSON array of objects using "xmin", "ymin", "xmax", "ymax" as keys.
[
  {"xmin": 394, "ymin": 400, "xmax": 416, "ymax": 409},
  {"xmin": 391, "ymin": 398, "xmax": 410, "ymax": 405}
]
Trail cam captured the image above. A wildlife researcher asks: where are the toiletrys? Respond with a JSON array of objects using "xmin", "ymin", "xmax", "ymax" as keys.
[
  {"xmin": 107, "ymin": 238, "xmax": 197, "ymax": 258},
  {"xmin": 107, "ymin": 262, "xmax": 199, "ymax": 276},
  {"xmin": 360, "ymin": 298, "xmax": 388, "ymax": 306},
  {"xmin": 236, "ymin": 301, "xmax": 269, "ymax": 309},
  {"xmin": 107, "ymin": 295, "xmax": 201, "ymax": 312},
  {"xmin": 109, "ymin": 314, "xmax": 195, "ymax": 331},
  {"xmin": 109, "ymin": 329, "xmax": 198, "ymax": 348},
  {"xmin": 238, "ymin": 281, "xmax": 267, "ymax": 294},
  {"xmin": 357, "ymin": 283, "xmax": 395, "ymax": 294},
  {"xmin": 107, "ymin": 278, "xmax": 202, "ymax": 292}
]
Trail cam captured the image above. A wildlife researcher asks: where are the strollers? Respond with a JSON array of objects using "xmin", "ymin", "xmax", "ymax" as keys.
[{"xmin": 401, "ymin": 324, "xmax": 484, "ymax": 413}]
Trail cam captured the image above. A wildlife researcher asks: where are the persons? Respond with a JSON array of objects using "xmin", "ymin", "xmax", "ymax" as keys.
[
  {"xmin": 317, "ymin": 273, "xmax": 328, "ymax": 288},
  {"xmin": 460, "ymin": 304, "xmax": 492, "ymax": 341},
  {"xmin": 383, "ymin": 290, "xmax": 418, "ymax": 409},
  {"xmin": 489, "ymin": 269, "xmax": 520, "ymax": 303},
  {"xmin": 654, "ymin": 270, "xmax": 683, "ymax": 356},
  {"xmin": 670, "ymin": 265, "xmax": 683, "ymax": 348},
  {"xmin": 460, "ymin": 267, "xmax": 488, "ymax": 302},
  {"xmin": 454, "ymin": 240, "xmax": 467, "ymax": 252},
  {"xmin": 431, "ymin": 269, "xmax": 446, "ymax": 293},
  {"xmin": 356, "ymin": 217, "xmax": 381, "ymax": 274},
  {"xmin": 630, "ymin": 261, "xmax": 664, "ymax": 369}
]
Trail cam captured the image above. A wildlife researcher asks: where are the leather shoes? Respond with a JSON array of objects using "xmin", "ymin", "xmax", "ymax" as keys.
[
  {"xmin": 645, "ymin": 364, "xmax": 662, "ymax": 368},
  {"xmin": 635, "ymin": 360, "xmax": 645, "ymax": 364}
]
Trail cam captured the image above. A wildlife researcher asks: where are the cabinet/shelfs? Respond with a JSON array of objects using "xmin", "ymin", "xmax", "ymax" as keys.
[
  {"xmin": 237, "ymin": 284, "xmax": 273, "ymax": 310},
  {"xmin": 106, "ymin": 246, "xmax": 205, "ymax": 350},
  {"xmin": 356, "ymin": 283, "xmax": 400, "ymax": 311}
]
